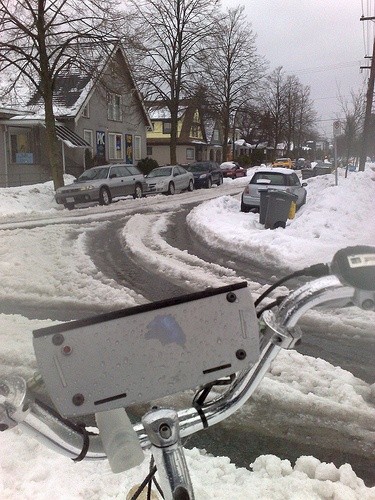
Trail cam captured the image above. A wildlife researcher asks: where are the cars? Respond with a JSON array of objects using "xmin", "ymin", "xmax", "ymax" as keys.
[
  {"xmin": 145, "ymin": 165, "xmax": 195, "ymax": 196},
  {"xmin": 241, "ymin": 167, "xmax": 308, "ymax": 212},
  {"xmin": 292, "ymin": 158, "xmax": 339, "ymax": 176},
  {"xmin": 220, "ymin": 161, "xmax": 246, "ymax": 180},
  {"xmin": 272, "ymin": 157, "xmax": 293, "ymax": 169},
  {"xmin": 55, "ymin": 163, "xmax": 147, "ymax": 211},
  {"xmin": 187, "ymin": 161, "xmax": 223, "ymax": 189}
]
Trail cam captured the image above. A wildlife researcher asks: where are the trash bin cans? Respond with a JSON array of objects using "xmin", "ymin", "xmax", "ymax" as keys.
[
  {"xmin": 258, "ymin": 188, "xmax": 281, "ymax": 224},
  {"xmin": 263, "ymin": 188, "xmax": 294, "ymax": 229}
]
